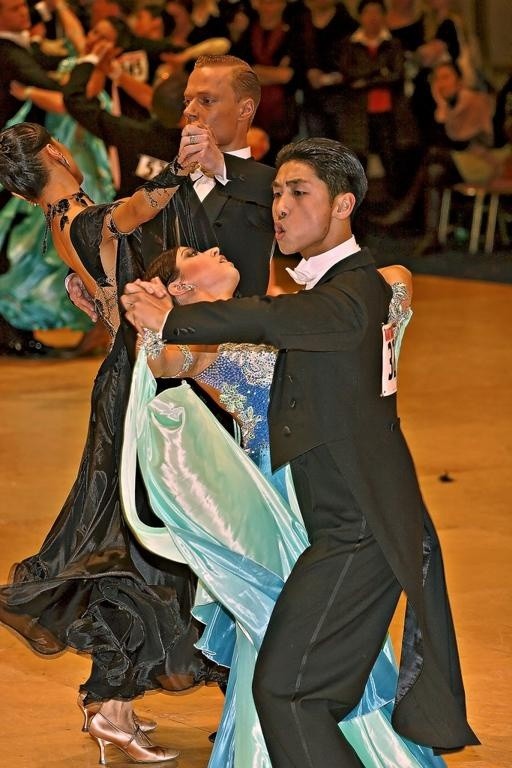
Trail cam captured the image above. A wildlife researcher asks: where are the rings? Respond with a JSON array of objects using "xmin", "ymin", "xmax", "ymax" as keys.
[{"xmin": 189, "ymin": 136, "xmax": 196, "ymax": 143}]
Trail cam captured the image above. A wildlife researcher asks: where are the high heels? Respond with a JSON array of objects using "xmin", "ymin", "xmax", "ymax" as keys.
[
  {"xmin": 77, "ymin": 690, "xmax": 158, "ymax": 733},
  {"xmin": 89, "ymin": 711, "xmax": 181, "ymax": 765}
]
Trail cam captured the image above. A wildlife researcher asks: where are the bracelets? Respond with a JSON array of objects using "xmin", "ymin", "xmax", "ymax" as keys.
[{"xmin": 136, "ymin": 330, "xmax": 164, "ymax": 361}]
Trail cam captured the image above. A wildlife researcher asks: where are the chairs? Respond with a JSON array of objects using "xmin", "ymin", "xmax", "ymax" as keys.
[
  {"xmin": 437, "ymin": 148, "xmax": 489, "ymax": 255},
  {"xmin": 480, "ymin": 144, "xmax": 511, "ymax": 257}
]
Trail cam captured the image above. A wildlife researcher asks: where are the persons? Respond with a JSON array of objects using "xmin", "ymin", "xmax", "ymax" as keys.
[
  {"xmin": 120, "ymin": 243, "xmax": 448, "ymax": 766},
  {"xmin": 62, "ymin": 53, "xmax": 278, "ymax": 744},
  {"xmin": 1, "ymin": 1, "xmax": 510, "ymax": 359},
  {"xmin": 120, "ymin": 135, "xmax": 481, "ymax": 766},
  {"xmin": 0, "ymin": 120, "xmax": 200, "ymax": 766}
]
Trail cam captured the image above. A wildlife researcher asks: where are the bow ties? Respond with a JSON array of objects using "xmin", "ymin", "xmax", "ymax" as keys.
[{"xmin": 285, "ymin": 258, "xmax": 316, "ymax": 285}]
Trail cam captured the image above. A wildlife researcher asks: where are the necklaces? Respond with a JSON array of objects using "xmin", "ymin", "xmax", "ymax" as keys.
[{"xmin": 33, "ymin": 189, "xmax": 86, "ymax": 225}]
